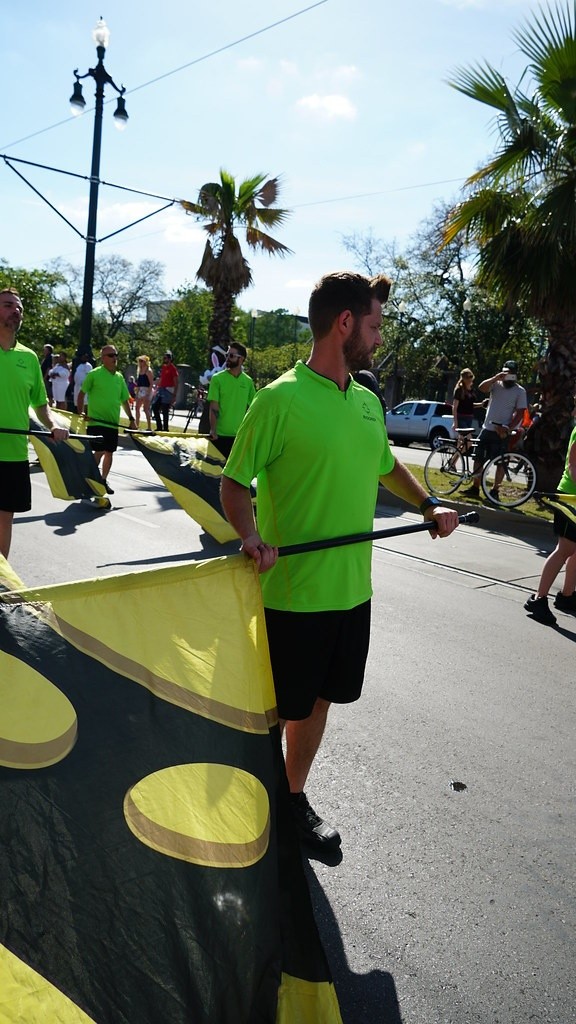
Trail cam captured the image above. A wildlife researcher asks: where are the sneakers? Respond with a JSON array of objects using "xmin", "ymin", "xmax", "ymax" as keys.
[
  {"xmin": 555, "ymin": 591, "xmax": 576, "ymax": 614},
  {"xmin": 524, "ymin": 593, "xmax": 557, "ymax": 624},
  {"xmin": 103, "ymin": 480, "xmax": 114, "ymax": 494},
  {"xmin": 289, "ymin": 791, "xmax": 342, "ymax": 852}
]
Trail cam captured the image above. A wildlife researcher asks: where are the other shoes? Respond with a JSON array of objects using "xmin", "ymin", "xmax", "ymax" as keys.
[
  {"xmin": 488, "ymin": 490, "xmax": 501, "ymax": 502},
  {"xmin": 458, "ymin": 486, "xmax": 480, "ymax": 497}
]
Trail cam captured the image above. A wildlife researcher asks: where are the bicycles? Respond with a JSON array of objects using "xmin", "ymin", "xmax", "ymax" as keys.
[
  {"xmin": 423, "ymin": 420, "xmax": 539, "ymax": 508},
  {"xmin": 183, "ymin": 383, "xmax": 209, "ymax": 434},
  {"xmin": 150, "ymin": 400, "xmax": 175, "ymax": 422}
]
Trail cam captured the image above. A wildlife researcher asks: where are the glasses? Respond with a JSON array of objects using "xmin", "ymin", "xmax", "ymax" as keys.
[
  {"xmin": 226, "ymin": 353, "xmax": 245, "ymax": 360},
  {"xmin": 139, "ymin": 361, "xmax": 143, "ymax": 363},
  {"xmin": 103, "ymin": 353, "xmax": 119, "ymax": 357},
  {"xmin": 467, "ymin": 376, "xmax": 473, "ymax": 378}
]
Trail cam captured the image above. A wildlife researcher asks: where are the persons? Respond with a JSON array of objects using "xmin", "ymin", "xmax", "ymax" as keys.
[
  {"xmin": 219, "ymin": 272, "xmax": 460, "ymax": 849},
  {"xmin": 76, "ymin": 344, "xmax": 138, "ymax": 494},
  {"xmin": 0, "ymin": 286, "xmax": 70, "ymax": 556},
  {"xmin": 152, "ymin": 351, "xmax": 179, "ymax": 432},
  {"xmin": 446, "ymin": 367, "xmax": 489, "ymax": 472},
  {"xmin": 458, "ymin": 358, "xmax": 527, "ymax": 503},
  {"xmin": 48, "ymin": 351, "xmax": 71, "ymax": 410},
  {"xmin": 206, "ymin": 342, "xmax": 256, "ymax": 459},
  {"xmin": 127, "ymin": 374, "xmax": 137, "ymax": 399},
  {"xmin": 134, "ymin": 355, "xmax": 154, "ymax": 431},
  {"xmin": 64, "ymin": 345, "xmax": 96, "ymax": 413},
  {"xmin": 41, "ymin": 344, "xmax": 53, "ymax": 407},
  {"xmin": 524, "ymin": 427, "xmax": 576, "ymax": 623}
]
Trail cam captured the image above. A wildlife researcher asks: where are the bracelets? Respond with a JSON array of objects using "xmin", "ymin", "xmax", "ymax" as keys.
[{"xmin": 129, "ymin": 416, "xmax": 134, "ymax": 421}]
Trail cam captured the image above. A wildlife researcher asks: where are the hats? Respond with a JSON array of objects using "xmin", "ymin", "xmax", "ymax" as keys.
[
  {"xmin": 503, "ymin": 360, "xmax": 518, "ymax": 372},
  {"xmin": 136, "ymin": 355, "xmax": 150, "ymax": 367}
]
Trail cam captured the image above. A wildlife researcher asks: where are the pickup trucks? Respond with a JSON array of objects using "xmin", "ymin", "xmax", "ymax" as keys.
[{"xmin": 386, "ymin": 400, "xmax": 484, "ymax": 454}]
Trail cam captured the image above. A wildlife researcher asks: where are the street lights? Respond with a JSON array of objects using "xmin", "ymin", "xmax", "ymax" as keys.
[{"xmin": 67, "ymin": 16, "xmax": 130, "ymax": 369}]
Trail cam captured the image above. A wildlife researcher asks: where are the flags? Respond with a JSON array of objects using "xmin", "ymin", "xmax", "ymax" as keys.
[
  {"xmin": 534, "ymin": 490, "xmax": 576, "ymax": 526},
  {"xmin": 132, "ymin": 433, "xmax": 258, "ymax": 544},
  {"xmin": 0, "ymin": 548, "xmax": 345, "ymax": 1024},
  {"xmin": 28, "ymin": 435, "xmax": 106, "ymax": 501}
]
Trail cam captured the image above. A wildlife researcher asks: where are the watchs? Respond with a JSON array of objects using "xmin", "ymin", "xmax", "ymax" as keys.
[{"xmin": 419, "ymin": 496, "xmax": 443, "ymax": 516}]
